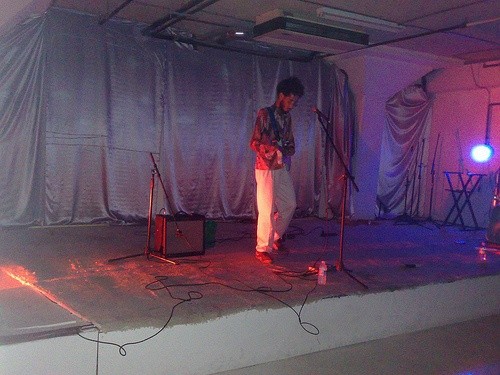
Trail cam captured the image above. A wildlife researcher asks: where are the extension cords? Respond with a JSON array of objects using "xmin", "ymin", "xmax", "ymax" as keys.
[{"xmin": 320, "ymin": 233, "xmax": 338, "ymax": 237}]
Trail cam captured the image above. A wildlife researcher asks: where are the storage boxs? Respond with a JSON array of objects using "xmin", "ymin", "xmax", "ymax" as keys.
[{"xmin": 154, "ymin": 214, "xmax": 205, "ymax": 258}]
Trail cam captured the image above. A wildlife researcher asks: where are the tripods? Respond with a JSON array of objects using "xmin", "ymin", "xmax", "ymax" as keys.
[
  {"xmin": 395, "ymin": 134, "xmax": 440, "ymax": 230},
  {"xmin": 108, "ymin": 152, "xmax": 179, "ymax": 265},
  {"xmin": 303, "ymin": 114, "xmax": 369, "ymax": 289}
]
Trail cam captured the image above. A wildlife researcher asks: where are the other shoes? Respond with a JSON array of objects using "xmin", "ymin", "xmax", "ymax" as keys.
[
  {"xmin": 269, "ymin": 240, "xmax": 287, "ymax": 254},
  {"xmin": 255, "ymin": 249, "xmax": 273, "ymax": 264}
]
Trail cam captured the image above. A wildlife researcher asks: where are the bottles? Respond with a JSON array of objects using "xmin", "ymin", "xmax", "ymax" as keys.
[
  {"xmin": 479, "ymin": 243, "xmax": 487, "ymax": 264},
  {"xmin": 317, "ymin": 261, "xmax": 327, "ymax": 286}
]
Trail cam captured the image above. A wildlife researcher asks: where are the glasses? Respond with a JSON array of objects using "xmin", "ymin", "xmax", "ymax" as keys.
[{"xmin": 285, "ymin": 96, "xmax": 298, "ymax": 107}]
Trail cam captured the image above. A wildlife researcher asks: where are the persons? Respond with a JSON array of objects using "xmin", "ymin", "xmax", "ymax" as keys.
[{"xmin": 248, "ymin": 75, "xmax": 306, "ymax": 264}]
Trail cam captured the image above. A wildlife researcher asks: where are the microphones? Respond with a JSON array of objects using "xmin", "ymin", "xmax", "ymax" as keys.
[
  {"xmin": 177, "ymin": 232, "xmax": 193, "ymax": 249},
  {"xmin": 312, "ymin": 106, "xmax": 330, "ymax": 124}
]
